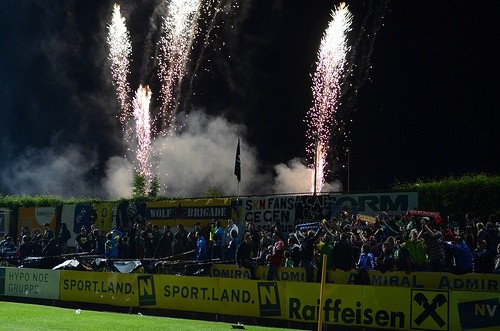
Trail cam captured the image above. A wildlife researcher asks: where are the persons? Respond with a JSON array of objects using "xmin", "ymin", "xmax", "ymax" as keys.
[{"xmin": 0, "ymin": 195, "xmax": 500, "ymax": 288}]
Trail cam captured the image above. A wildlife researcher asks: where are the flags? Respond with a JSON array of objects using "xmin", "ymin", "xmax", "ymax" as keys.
[{"xmin": 233, "ymin": 136, "xmax": 241, "ymax": 184}]
